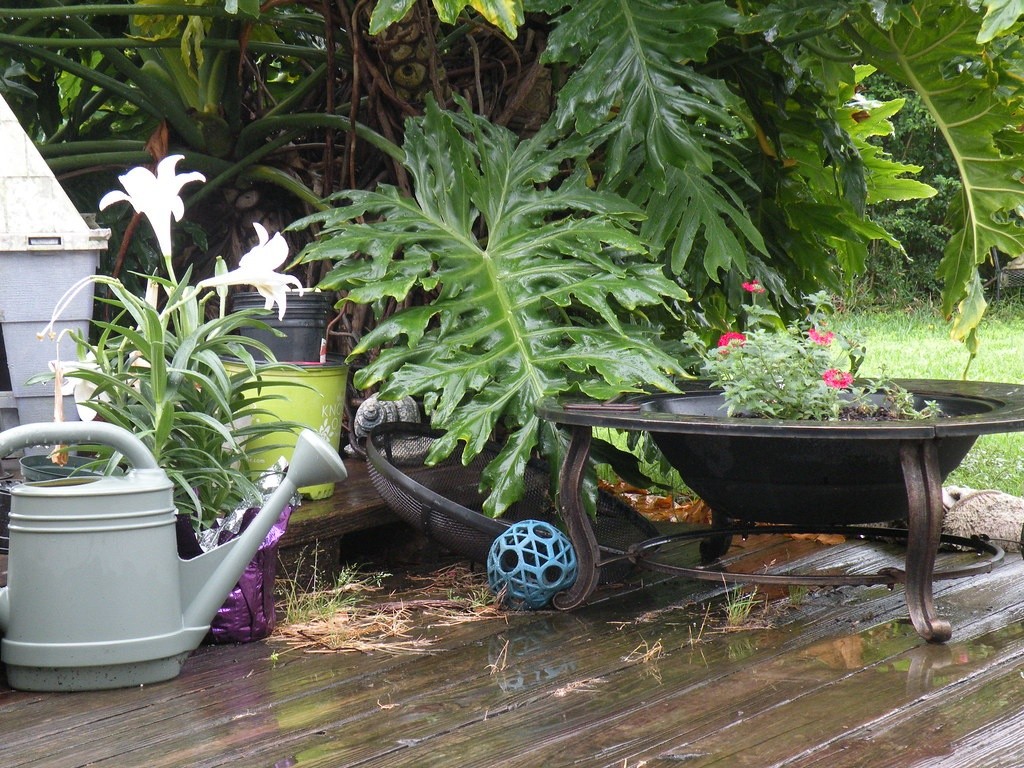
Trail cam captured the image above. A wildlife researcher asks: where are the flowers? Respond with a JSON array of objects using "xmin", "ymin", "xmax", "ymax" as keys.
[
  {"xmin": 680, "ymin": 277, "xmax": 948, "ymax": 421},
  {"xmin": 23, "ymin": 152, "xmax": 326, "ymax": 536}
]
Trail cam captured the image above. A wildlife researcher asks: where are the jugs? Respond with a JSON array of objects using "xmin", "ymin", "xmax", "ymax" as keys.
[{"xmin": 0, "ymin": 421, "xmax": 346, "ymax": 693}]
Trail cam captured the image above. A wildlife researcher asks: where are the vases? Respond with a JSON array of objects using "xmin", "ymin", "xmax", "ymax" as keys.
[
  {"xmin": 231, "ymin": 293, "xmax": 329, "ymax": 366},
  {"xmin": 20, "ymin": 455, "xmax": 123, "ymax": 484},
  {"xmin": 220, "ymin": 356, "xmax": 347, "ymax": 500},
  {"xmin": 532, "ymin": 378, "xmax": 1024, "ymax": 642}
]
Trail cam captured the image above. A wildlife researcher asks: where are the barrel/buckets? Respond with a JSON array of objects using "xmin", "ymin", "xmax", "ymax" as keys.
[
  {"xmin": 18, "ymin": 454, "xmax": 123, "ymax": 483},
  {"xmin": 215, "ymin": 289, "xmax": 351, "ymax": 499}
]
